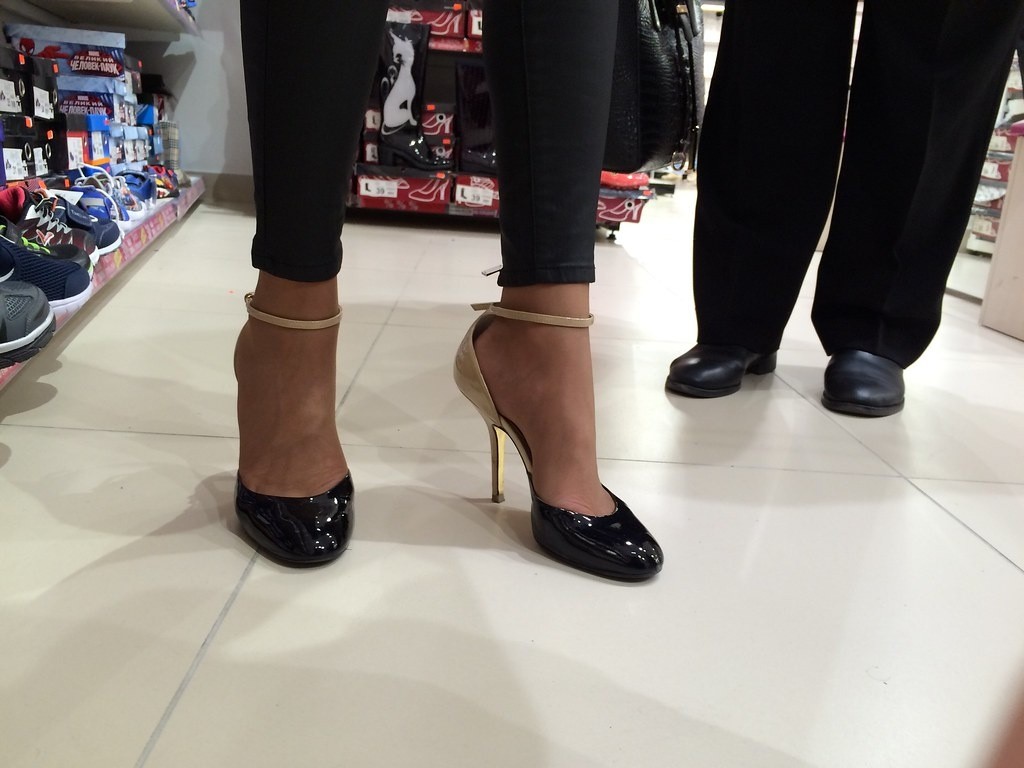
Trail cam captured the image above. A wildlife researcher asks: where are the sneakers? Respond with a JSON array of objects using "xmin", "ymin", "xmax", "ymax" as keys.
[{"xmin": 0, "ymin": 164, "xmax": 180, "ymax": 370}]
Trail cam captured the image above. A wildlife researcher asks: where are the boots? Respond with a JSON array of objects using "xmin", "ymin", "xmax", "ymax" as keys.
[{"xmin": 159, "ymin": 119, "xmax": 192, "ymax": 187}]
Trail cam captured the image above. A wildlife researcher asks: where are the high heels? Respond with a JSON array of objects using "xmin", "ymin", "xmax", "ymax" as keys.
[
  {"xmin": 451, "ymin": 304, "xmax": 665, "ymax": 583},
  {"xmin": 230, "ymin": 291, "xmax": 354, "ymax": 565},
  {"xmin": 379, "ymin": 120, "xmax": 454, "ymax": 171}
]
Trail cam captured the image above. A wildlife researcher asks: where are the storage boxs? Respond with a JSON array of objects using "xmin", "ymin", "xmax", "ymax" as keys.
[
  {"xmin": 0, "ymin": 23, "xmax": 164, "ymax": 185},
  {"xmin": 355, "ymin": 0, "xmax": 656, "ymax": 240}
]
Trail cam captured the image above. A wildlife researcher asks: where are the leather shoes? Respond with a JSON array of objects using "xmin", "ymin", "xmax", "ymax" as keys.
[
  {"xmin": 665, "ymin": 342, "xmax": 775, "ymax": 400},
  {"xmin": 458, "ymin": 142, "xmax": 501, "ymax": 176},
  {"xmin": 821, "ymin": 347, "xmax": 905, "ymax": 416}
]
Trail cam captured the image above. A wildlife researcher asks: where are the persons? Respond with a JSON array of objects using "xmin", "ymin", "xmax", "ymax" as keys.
[
  {"xmin": 234, "ymin": 0, "xmax": 664, "ymax": 582},
  {"xmin": 664, "ymin": 0, "xmax": 1024, "ymax": 417}
]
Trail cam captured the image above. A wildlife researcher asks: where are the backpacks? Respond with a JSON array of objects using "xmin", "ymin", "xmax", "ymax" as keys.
[{"xmin": 600, "ymin": 0, "xmax": 703, "ymax": 174}]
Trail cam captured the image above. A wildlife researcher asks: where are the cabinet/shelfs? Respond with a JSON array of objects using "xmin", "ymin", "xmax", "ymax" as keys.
[
  {"xmin": 0, "ymin": 0, "xmax": 206, "ymax": 389},
  {"xmin": 343, "ymin": 36, "xmax": 621, "ymax": 240}
]
